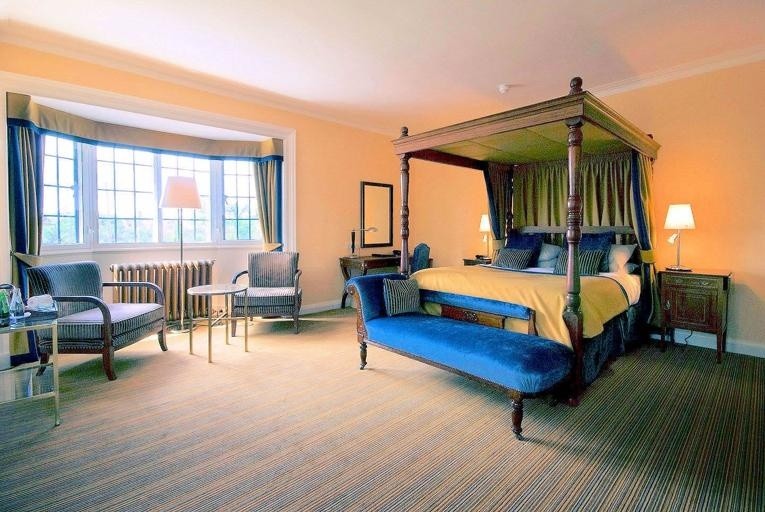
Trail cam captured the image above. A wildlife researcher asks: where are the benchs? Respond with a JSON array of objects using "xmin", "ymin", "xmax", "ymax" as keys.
[{"xmin": 345, "ymin": 273, "xmax": 573, "ymax": 440}]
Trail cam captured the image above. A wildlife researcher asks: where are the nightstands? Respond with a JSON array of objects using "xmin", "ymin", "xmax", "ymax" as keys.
[
  {"xmin": 656, "ymin": 270, "xmax": 734, "ymax": 364},
  {"xmin": 463, "ymin": 259, "xmax": 491, "ymax": 266}
]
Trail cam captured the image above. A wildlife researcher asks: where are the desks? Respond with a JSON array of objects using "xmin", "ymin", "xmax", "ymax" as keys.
[{"xmin": 339, "ymin": 255, "xmax": 434, "ymax": 309}]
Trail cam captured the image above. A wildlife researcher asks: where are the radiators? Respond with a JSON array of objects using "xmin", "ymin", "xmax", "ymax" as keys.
[{"xmin": 109, "ymin": 258, "xmax": 214, "ymax": 326}]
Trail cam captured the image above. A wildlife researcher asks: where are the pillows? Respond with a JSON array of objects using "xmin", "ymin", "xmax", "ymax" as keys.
[
  {"xmin": 383, "ymin": 278, "xmax": 422, "ymax": 317},
  {"xmin": 492, "ymin": 229, "xmax": 640, "ymax": 276}
]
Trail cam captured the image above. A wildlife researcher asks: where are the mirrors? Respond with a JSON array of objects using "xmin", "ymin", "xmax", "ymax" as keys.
[{"xmin": 360, "ymin": 180, "xmax": 393, "ymax": 248}]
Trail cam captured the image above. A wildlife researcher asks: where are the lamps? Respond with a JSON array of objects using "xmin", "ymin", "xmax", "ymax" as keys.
[
  {"xmin": 351, "ymin": 226, "xmax": 377, "ymax": 256},
  {"xmin": 158, "ymin": 176, "xmax": 202, "ymax": 331},
  {"xmin": 664, "ymin": 204, "xmax": 696, "ymax": 271},
  {"xmin": 480, "ymin": 214, "xmax": 492, "ymax": 257}
]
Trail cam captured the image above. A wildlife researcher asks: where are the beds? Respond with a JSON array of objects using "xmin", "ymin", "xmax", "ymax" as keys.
[{"xmin": 390, "ymin": 74, "xmax": 663, "ymax": 406}]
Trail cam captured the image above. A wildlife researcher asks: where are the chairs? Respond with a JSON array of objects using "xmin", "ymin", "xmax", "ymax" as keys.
[
  {"xmin": 22, "ymin": 261, "xmax": 168, "ymax": 381},
  {"xmin": 230, "ymin": 250, "xmax": 303, "ymax": 338},
  {"xmin": 410, "ymin": 242, "xmax": 430, "ymax": 274}
]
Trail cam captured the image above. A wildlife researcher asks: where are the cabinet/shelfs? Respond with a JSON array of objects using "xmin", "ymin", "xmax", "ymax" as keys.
[{"xmin": 0, "ymin": 317, "xmax": 61, "ymax": 426}]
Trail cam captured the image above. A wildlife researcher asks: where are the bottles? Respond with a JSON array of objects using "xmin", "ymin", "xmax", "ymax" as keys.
[
  {"xmin": 0, "ymin": 289, "xmax": 11, "ymax": 327},
  {"xmin": 8, "ymin": 288, "xmax": 26, "ymax": 328}
]
